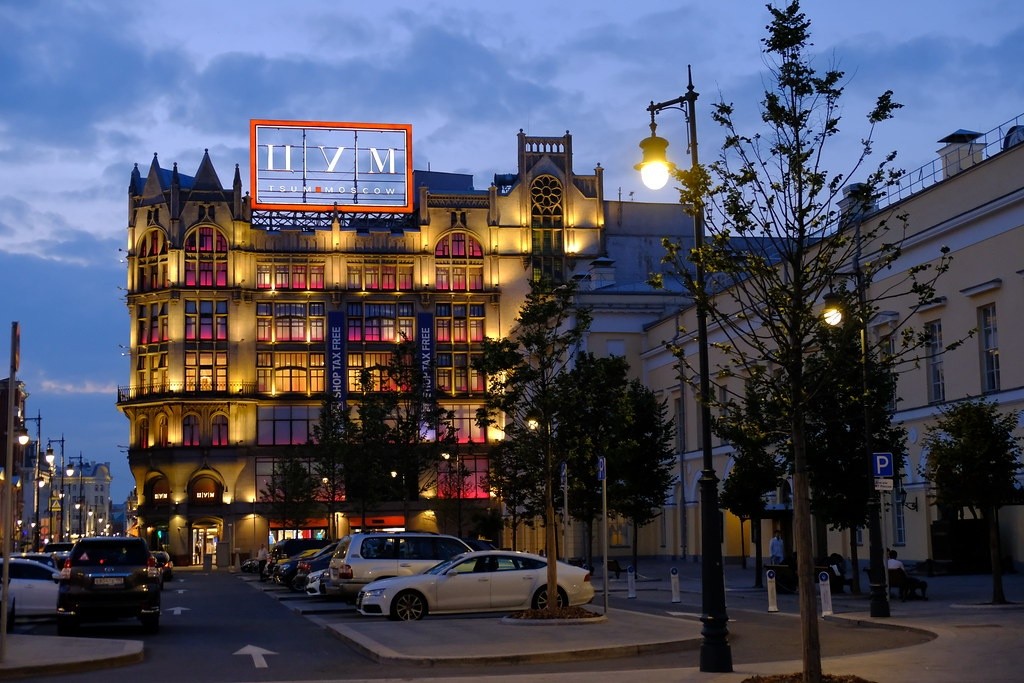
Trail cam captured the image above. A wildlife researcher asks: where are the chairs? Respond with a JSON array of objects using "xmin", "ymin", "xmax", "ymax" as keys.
[
  {"xmin": 473, "ymin": 558, "xmax": 499, "ymax": 572},
  {"xmin": 385, "ymin": 544, "xmax": 394, "ymax": 559},
  {"xmin": 376, "ymin": 541, "xmax": 386, "ymax": 558}
]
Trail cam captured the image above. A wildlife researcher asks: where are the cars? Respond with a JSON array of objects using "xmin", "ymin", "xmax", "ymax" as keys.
[
  {"xmin": 0, "ymin": 558, "xmax": 63, "ymax": 617},
  {"xmin": 43, "ymin": 542, "xmax": 74, "ymax": 569},
  {"xmin": 240, "ymin": 537, "xmax": 338, "ymax": 594},
  {"xmin": 149, "ymin": 550, "xmax": 174, "ymax": 582},
  {"xmin": 10, "ymin": 551, "xmax": 58, "ymax": 569},
  {"xmin": 0, "ymin": 559, "xmax": 17, "ymax": 635},
  {"xmin": 355, "ymin": 550, "xmax": 596, "ymax": 622}
]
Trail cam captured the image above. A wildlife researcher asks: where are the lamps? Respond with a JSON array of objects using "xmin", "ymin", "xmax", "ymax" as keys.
[
  {"xmin": 426, "ymin": 440, "xmax": 431, "ymax": 443},
  {"xmin": 495, "ymin": 245, "xmax": 498, "ymax": 249},
  {"xmin": 241, "ymin": 240, "xmax": 246, "ymax": 245},
  {"xmin": 226, "ymin": 503, "xmax": 231, "ymax": 506},
  {"xmin": 121, "ymin": 352, "xmax": 130, "ymax": 356},
  {"xmin": 424, "ymin": 245, "xmax": 428, "ymax": 249},
  {"xmin": 425, "ymin": 284, "xmax": 429, "ymax": 286},
  {"xmin": 495, "ymin": 439, "xmax": 499, "ymax": 443},
  {"xmin": 167, "ymin": 442, "xmax": 172, "ymax": 446},
  {"xmin": 524, "ymin": 250, "xmax": 528, "ymax": 253},
  {"xmin": 601, "ymin": 225, "xmax": 606, "ymax": 228},
  {"xmin": 493, "ymin": 497, "xmax": 497, "ymax": 500},
  {"xmin": 175, "ymin": 501, "xmax": 179, "ymax": 505},
  {"xmin": 169, "ymin": 390, "xmax": 174, "ymax": 395},
  {"xmin": 336, "ymin": 243, "xmax": 339, "ymax": 247},
  {"xmin": 120, "ymin": 259, "xmax": 128, "ymax": 264},
  {"xmin": 495, "ymin": 284, "xmax": 499, "ymax": 287},
  {"xmin": 239, "ymin": 440, "xmax": 243, "ymax": 445}
]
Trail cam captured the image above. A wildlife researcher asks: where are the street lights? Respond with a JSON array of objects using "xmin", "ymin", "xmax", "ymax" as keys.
[
  {"xmin": 17, "ymin": 409, "xmax": 42, "ymax": 552},
  {"xmin": 65, "ymin": 451, "xmax": 84, "ymax": 538},
  {"xmin": 814, "ymin": 260, "xmax": 893, "ymax": 618},
  {"xmin": 632, "ymin": 63, "xmax": 734, "ymax": 673},
  {"xmin": 45, "ymin": 432, "xmax": 66, "ymax": 542},
  {"xmin": 72, "ymin": 491, "xmax": 107, "ymax": 537}
]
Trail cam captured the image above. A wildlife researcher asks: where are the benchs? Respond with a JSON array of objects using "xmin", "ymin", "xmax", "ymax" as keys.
[
  {"xmin": 863, "ymin": 567, "xmax": 926, "ymax": 603},
  {"xmin": 764, "ymin": 563, "xmax": 854, "ymax": 595},
  {"xmin": 520, "ymin": 561, "xmax": 540, "ymax": 570}
]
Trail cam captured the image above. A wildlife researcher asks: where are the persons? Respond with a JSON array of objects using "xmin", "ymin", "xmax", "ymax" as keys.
[
  {"xmin": 258, "ymin": 544, "xmax": 267, "ymax": 581},
  {"xmin": 769, "ymin": 530, "xmax": 784, "ymax": 565},
  {"xmin": 194, "ymin": 542, "xmax": 201, "ymax": 564},
  {"xmin": 887, "ymin": 548, "xmax": 928, "ymax": 601},
  {"xmin": 540, "ymin": 550, "xmax": 543, "ymax": 556}
]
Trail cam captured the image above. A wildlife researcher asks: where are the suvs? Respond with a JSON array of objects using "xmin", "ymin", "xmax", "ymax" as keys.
[
  {"xmin": 56, "ymin": 536, "xmax": 166, "ymax": 636},
  {"xmin": 319, "ymin": 530, "xmax": 523, "ymax": 607}
]
